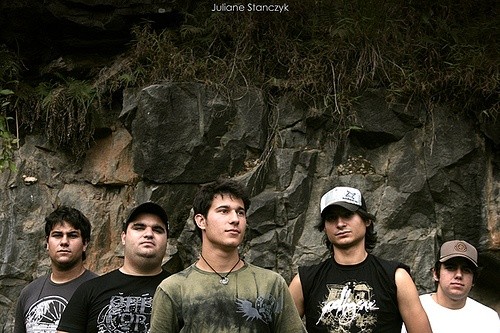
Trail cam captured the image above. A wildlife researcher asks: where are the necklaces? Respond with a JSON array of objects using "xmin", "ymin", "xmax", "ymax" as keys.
[{"xmin": 201, "ymin": 254, "xmax": 240, "ymax": 285}]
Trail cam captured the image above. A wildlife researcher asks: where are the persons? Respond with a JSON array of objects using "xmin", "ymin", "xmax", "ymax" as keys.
[
  {"xmin": 56, "ymin": 202, "xmax": 185, "ymax": 333},
  {"xmin": 401, "ymin": 240, "xmax": 500, "ymax": 333},
  {"xmin": 14, "ymin": 207, "xmax": 100, "ymax": 333},
  {"xmin": 149, "ymin": 180, "xmax": 308, "ymax": 333},
  {"xmin": 289, "ymin": 186, "xmax": 432, "ymax": 333}
]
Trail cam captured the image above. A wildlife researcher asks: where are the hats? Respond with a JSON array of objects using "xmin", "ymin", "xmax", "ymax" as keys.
[
  {"xmin": 122, "ymin": 202, "xmax": 170, "ymax": 237},
  {"xmin": 435, "ymin": 239, "xmax": 478, "ymax": 267},
  {"xmin": 320, "ymin": 186, "xmax": 367, "ymax": 213}
]
